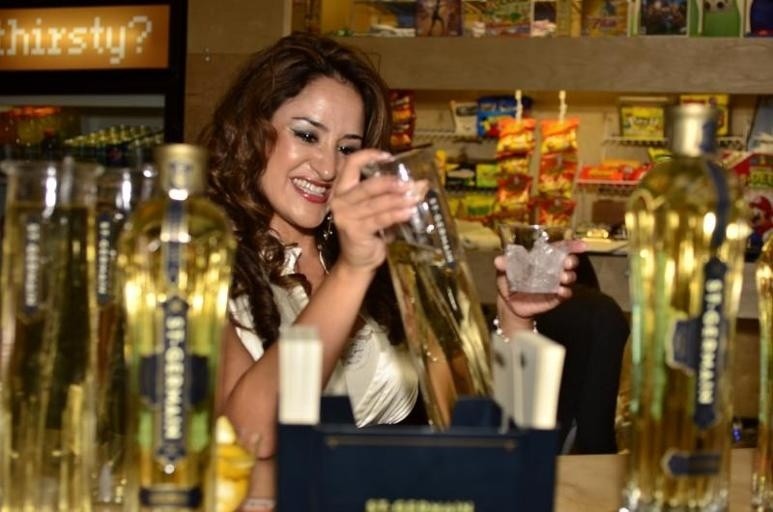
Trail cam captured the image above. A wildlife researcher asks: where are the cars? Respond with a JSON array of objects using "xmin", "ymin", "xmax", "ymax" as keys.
[
  {"xmin": 497, "ymin": 221, "xmax": 572, "ymax": 295},
  {"xmin": 371, "ymin": 147, "xmax": 491, "ymax": 421}
]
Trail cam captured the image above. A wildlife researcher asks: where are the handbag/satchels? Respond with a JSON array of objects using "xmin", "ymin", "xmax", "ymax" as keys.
[{"xmin": 492, "ymin": 316, "xmax": 540, "ymax": 344}]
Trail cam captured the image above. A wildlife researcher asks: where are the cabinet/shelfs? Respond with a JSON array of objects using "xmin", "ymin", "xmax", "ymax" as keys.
[
  {"xmin": 0, "ymin": 106, "xmax": 161, "ymax": 161},
  {"xmin": 113, "ymin": 137, "xmax": 234, "ymax": 509},
  {"xmin": 612, "ymin": 102, "xmax": 749, "ymax": 511}
]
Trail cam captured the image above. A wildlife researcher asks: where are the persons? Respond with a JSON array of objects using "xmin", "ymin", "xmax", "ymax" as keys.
[
  {"xmin": 182, "ymin": 27, "xmax": 582, "ymax": 469},
  {"xmin": 531, "ymin": 253, "xmax": 630, "ymax": 454}
]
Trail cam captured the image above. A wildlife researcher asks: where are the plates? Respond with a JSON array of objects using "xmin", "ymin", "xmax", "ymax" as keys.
[{"xmin": 306, "ymin": 2, "xmax": 772, "ymax": 320}]
